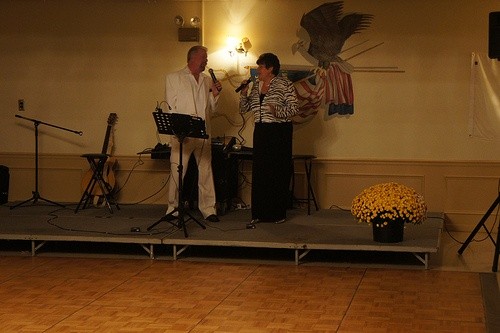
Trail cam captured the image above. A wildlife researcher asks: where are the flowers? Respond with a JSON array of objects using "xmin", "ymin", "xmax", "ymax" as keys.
[{"xmin": 351, "ymin": 183, "xmax": 427, "ymax": 227}]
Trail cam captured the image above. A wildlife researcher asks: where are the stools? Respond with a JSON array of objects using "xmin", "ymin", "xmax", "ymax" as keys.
[
  {"xmin": 74, "ymin": 154, "xmax": 120, "ymax": 214},
  {"xmin": 289, "ymin": 155, "xmax": 319, "ymax": 216}
]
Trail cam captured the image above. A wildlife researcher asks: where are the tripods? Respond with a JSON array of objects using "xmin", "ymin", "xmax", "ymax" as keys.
[
  {"xmin": 147, "ymin": 112, "xmax": 209, "ymax": 239},
  {"xmin": 9, "ymin": 114, "xmax": 83, "ymax": 211}
]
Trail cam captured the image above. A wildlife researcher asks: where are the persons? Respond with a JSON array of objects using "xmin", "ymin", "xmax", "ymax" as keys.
[
  {"xmin": 239, "ymin": 53, "xmax": 299, "ymax": 224},
  {"xmin": 160, "ymin": 45, "xmax": 222, "ymax": 222}
]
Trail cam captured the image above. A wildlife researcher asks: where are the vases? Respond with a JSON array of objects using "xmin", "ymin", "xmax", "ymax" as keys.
[{"xmin": 373, "ymin": 212, "xmax": 405, "ymax": 243}]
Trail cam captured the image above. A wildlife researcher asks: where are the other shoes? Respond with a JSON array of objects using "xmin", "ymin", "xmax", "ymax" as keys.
[
  {"xmin": 273, "ymin": 216, "xmax": 285, "ymax": 224},
  {"xmin": 162, "ymin": 214, "xmax": 179, "ymax": 220},
  {"xmin": 251, "ymin": 217, "xmax": 263, "ymax": 223},
  {"xmin": 207, "ymin": 215, "xmax": 219, "ymax": 222}
]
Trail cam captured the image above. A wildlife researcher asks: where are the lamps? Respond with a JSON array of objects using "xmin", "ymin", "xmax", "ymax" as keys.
[
  {"xmin": 226, "ymin": 37, "xmax": 252, "ymax": 56},
  {"xmin": 174, "ymin": 14, "xmax": 200, "ymax": 43}
]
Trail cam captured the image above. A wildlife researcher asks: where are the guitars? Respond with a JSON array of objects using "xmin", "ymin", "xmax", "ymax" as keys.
[{"xmin": 80, "ymin": 112, "xmax": 119, "ymax": 195}]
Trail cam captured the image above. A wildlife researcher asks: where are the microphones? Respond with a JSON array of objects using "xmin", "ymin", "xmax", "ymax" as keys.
[
  {"xmin": 209, "ymin": 68, "xmax": 221, "ymax": 92},
  {"xmin": 234, "ymin": 76, "xmax": 255, "ymax": 93},
  {"xmin": 232, "ymin": 144, "xmax": 254, "ymax": 153}
]
